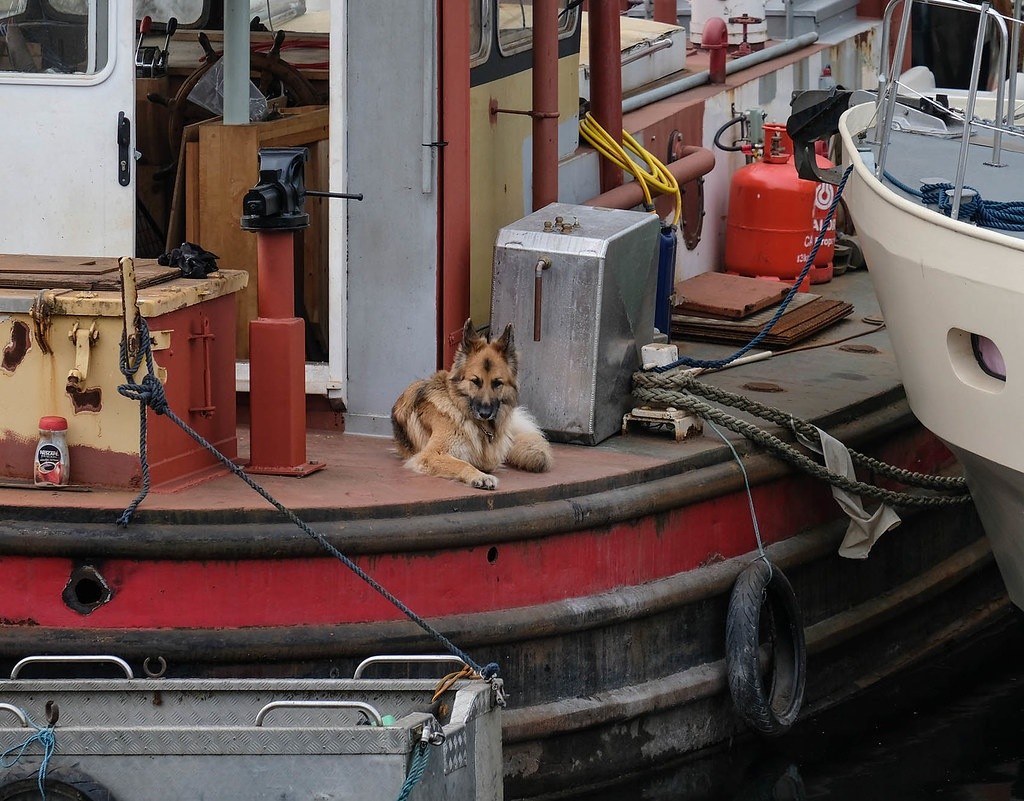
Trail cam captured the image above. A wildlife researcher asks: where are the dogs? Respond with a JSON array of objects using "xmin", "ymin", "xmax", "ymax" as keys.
[{"xmin": 389, "ymin": 316, "xmax": 555, "ymax": 492}]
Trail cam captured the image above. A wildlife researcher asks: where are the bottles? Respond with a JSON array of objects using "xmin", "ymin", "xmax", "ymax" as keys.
[{"xmin": 32, "ymin": 416, "xmax": 70, "ymax": 487}]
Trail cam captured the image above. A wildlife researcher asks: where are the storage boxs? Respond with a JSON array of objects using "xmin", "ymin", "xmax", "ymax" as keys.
[
  {"xmin": 490, "ymin": 202, "xmax": 662, "ymax": 446},
  {"xmin": 1, "ymin": 254, "xmax": 251, "ymax": 490}
]
